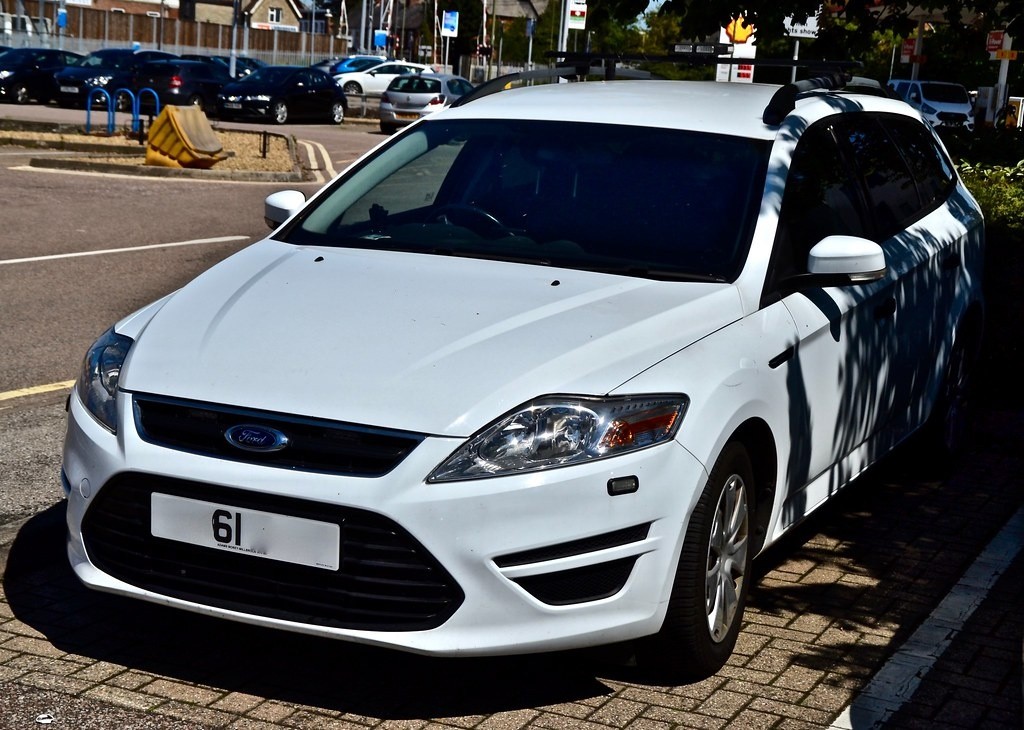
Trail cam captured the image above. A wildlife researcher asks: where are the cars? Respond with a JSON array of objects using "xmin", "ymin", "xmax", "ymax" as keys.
[
  {"xmin": 62, "ymin": 78, "xmax": 994, "ymax": 678},
  {"xmin": 0, "ymin": 44, "xmax": 670, "ymax": 137}
]
